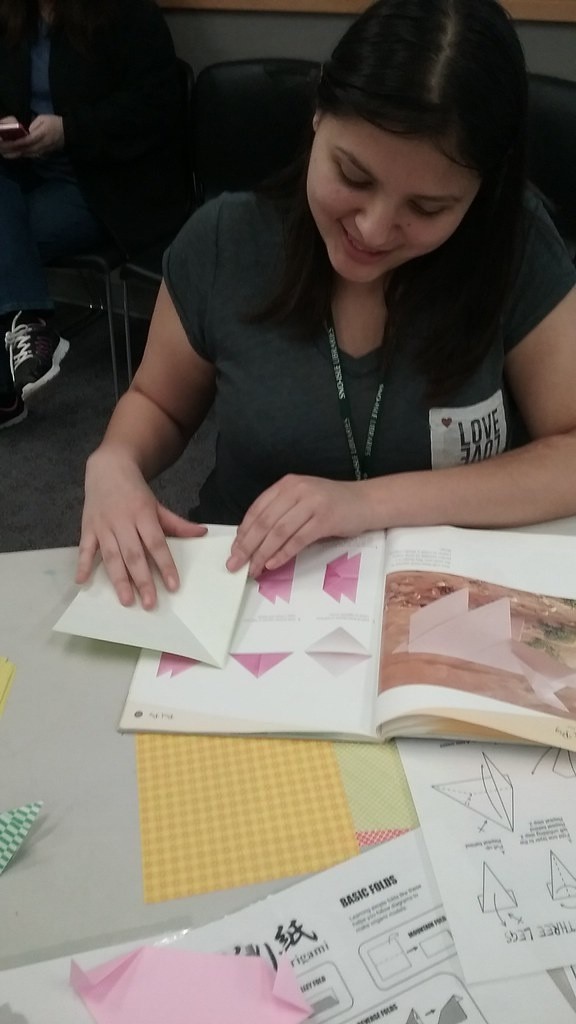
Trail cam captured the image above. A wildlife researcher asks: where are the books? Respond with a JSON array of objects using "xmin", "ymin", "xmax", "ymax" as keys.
[{"xmin": 116, "ymin": 524, "xmax": 576, "ymax": 751}]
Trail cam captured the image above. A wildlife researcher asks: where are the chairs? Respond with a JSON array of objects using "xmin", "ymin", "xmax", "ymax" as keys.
[{"xmin": 49, "ymin": 54, "xmax": 576, "ymax": 410}]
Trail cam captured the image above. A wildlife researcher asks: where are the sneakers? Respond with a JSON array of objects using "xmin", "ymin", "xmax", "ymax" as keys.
[
  {"xmin": 5, "ymin": 310, "xmax": 69, "ymax": 407},
  {"xmin": 1, "ymin": 387, "xmax": 27, "ymax": 429}
]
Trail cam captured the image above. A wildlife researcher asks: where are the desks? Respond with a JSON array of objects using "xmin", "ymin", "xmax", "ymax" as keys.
[{"xmin": 0, "ymin": 512, "xmax": 576, "ymax": 1024}]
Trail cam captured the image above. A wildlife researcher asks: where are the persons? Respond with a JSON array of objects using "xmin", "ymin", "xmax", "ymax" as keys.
[
  {"xmin": 0, "ymin": 0, "xmax": 178, "ymax": 429},
  {"xmin": 75, "ymin": 0, "xmax": 576, "ymax": 610}
]
